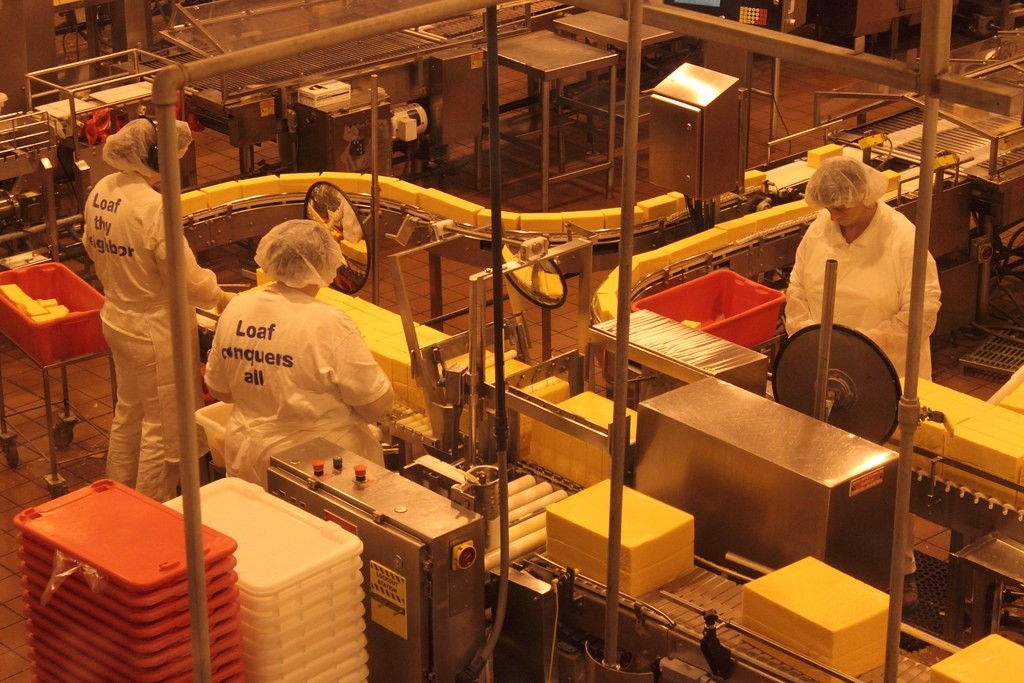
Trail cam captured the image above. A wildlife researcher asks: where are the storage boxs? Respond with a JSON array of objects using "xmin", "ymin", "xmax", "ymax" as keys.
[
  {"xmin": 632, "ymin": 268, "xmax": 786, "ymax": 349},
  {"xmin": 0, "ymin": 262, "xmax": 107, "ymax": 365}
]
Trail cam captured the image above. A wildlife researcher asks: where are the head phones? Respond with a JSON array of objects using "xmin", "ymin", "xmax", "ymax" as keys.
[{"xmin": 138, "ymin": 113, "xmax": 160, "ymax": 171}]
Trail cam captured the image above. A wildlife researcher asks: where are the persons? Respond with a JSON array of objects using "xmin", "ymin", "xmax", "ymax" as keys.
[
  {"xmin": 82, "ymin": 115, "xmax": 242, "ymax": 504},
  {"xmin": 783, "ymin": 156, "xmax": 942, "ymax": 382},
  {"xmin": 202, "ymin": 218, "xmax": 395, "ymax": 495}
]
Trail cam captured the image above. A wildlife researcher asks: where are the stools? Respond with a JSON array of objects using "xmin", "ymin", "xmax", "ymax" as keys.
[
  {"xmin": 476, "ymin": 30, "xmax": 619, "ymax": 212},
  {"xmin": 553, "ymin": 10, "xmax": 691, "ymax": 174}
]
